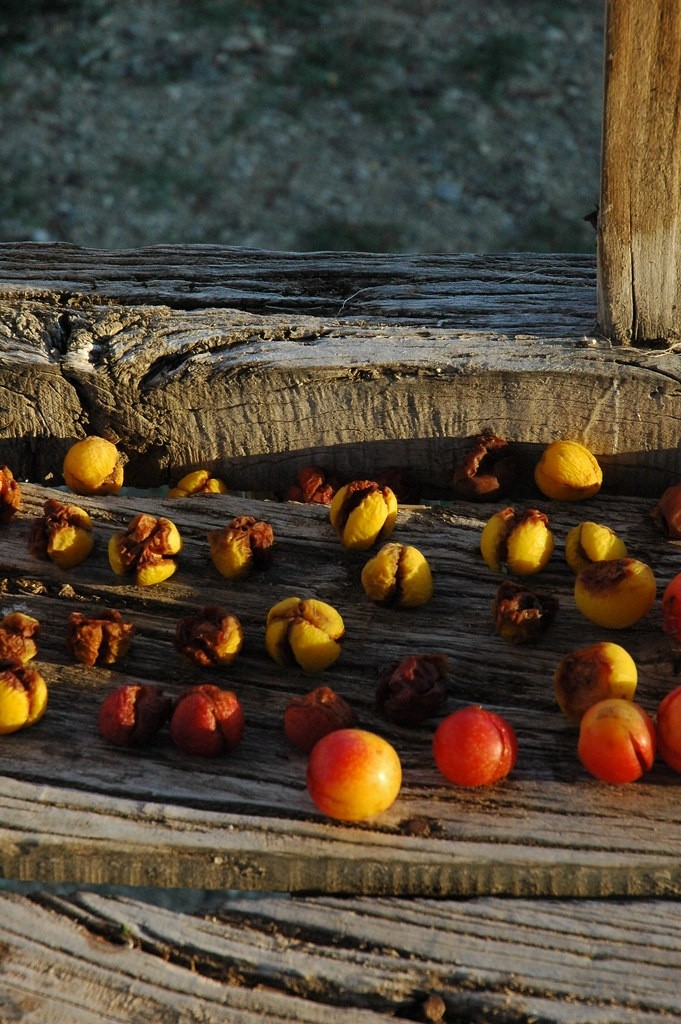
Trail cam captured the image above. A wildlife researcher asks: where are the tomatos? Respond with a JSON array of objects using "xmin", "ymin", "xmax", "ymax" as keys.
[{"xmin": 306, "ymin": 686, "xmax": 681, "ymax": 822}]
